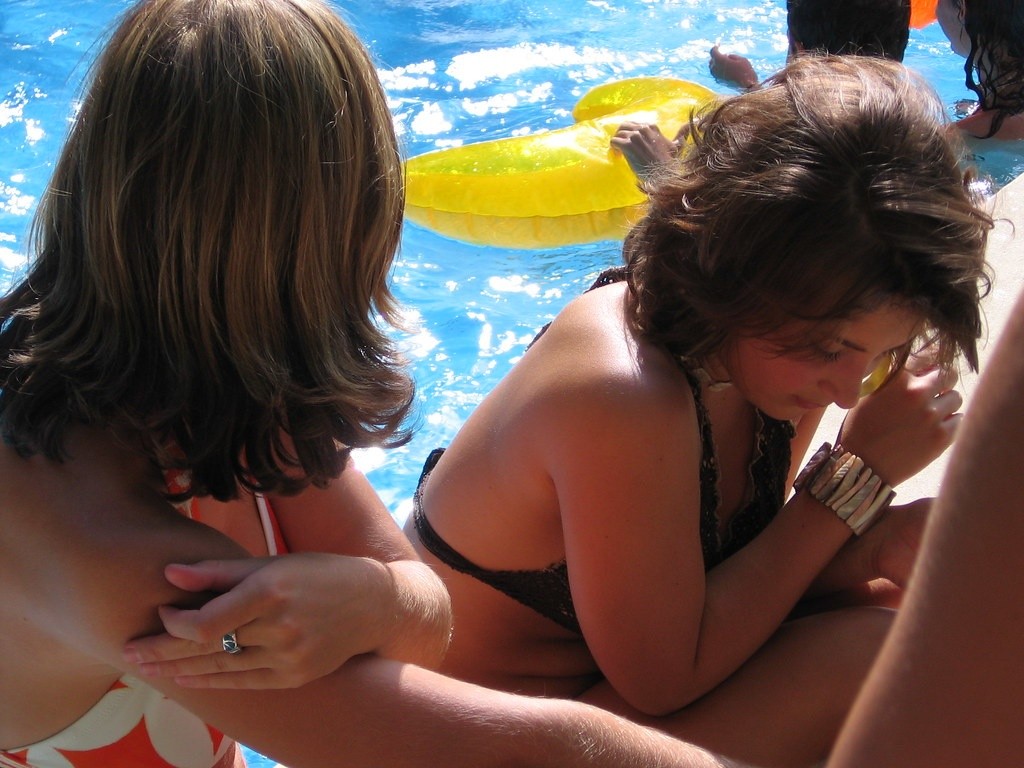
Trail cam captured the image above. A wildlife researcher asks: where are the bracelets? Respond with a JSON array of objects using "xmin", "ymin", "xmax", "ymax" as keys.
[{"xmin": 793, "ymin": 442, "xmax": 895, "ymax": 536}]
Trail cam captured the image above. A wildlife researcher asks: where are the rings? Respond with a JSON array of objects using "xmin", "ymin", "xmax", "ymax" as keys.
[{"xmin": 222, "ymin": 627, "xmax": 241, "ymax": 655}]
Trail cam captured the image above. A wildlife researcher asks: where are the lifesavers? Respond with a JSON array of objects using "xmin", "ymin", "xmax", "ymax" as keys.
[{"xmin": 398, "ymin": 77, "xmax": 723, "ymax": 249}]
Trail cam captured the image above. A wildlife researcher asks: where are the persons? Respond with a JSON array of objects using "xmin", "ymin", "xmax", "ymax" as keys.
[
  {"xmin": 403, "ymin": 37, "xmax": 1001, "ymax": 768},
  {"xmin": 710, "ymin": 0, "xmax": 1023, "ymax": 152},
  {"xmin": 0, "ymin": 0, "xmax": 762, "ymax": 768},
  {"xmin": 609, "ymin": 1, "xmax": 912, "ymax": 180},
  {"xmin": 814, "ymin": 289, "xmax": 1024, "ymax": 765}
]
